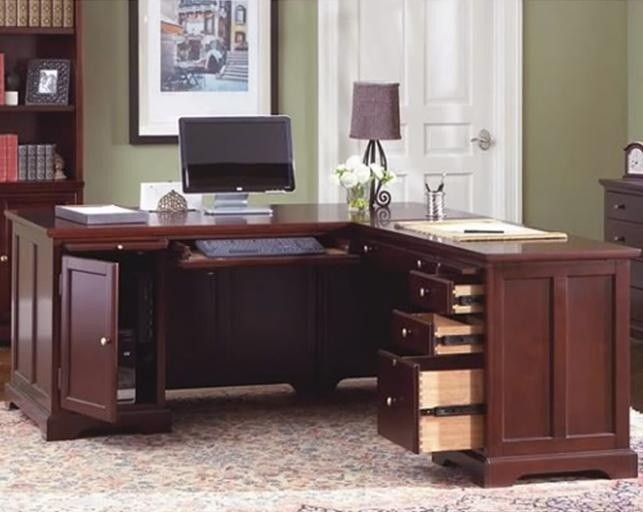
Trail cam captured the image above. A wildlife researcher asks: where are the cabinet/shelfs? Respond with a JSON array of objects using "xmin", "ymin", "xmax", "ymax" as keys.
[
  {"xmin": 598, "ymin": 178, "xmax": 643, "ymax": 413},
  {"xmin": 1, "ymin": 0, "xmax": 85, "ymax": 343}
]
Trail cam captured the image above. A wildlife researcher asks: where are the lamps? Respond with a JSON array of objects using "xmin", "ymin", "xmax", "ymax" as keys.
[{"xmin": 349, "ymin": 81, "xmax": 403, "ymax": 210}]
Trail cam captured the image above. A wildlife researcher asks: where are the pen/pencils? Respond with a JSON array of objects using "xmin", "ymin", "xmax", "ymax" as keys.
[{"xmin": 465, "ymin": 229, "xmax": 504, "ymax": 234}]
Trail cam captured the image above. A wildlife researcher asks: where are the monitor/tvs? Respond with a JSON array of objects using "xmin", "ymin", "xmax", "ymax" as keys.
[{"xmin": 178, "ymin": 115, "xmax": 296, "ymax": 215}]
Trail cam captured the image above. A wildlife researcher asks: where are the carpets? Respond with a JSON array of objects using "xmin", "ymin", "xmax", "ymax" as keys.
[{"xmin": 0, "ymin": 376, "xmax": 642, "ymax": 512}]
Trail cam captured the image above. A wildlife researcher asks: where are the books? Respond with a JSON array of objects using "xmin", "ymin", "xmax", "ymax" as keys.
[
  {"xmin": 55, "ymin": 202, "xmax": 145, "ymax": 225},
  {"xmin": 0, "ymin": 133, "xmax": 55, "ymax": 183},
  {"xmin": 0, "ymin": 1, "xmax": 74, "ymax": 28}
]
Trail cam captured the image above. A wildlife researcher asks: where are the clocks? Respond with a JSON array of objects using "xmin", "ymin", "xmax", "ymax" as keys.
[{"xmin": 622, "ymin": 140, "xmax": 643, "ymax": 180}]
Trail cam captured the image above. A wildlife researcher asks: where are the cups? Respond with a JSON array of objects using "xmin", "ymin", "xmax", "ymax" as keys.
[
  {"xmin": 426, "ymin": 191, "xmax": 445, "ymax": 218},
  {"xmin": 5, "ymin": 91, "xmax": 19, "ymax": 106}
]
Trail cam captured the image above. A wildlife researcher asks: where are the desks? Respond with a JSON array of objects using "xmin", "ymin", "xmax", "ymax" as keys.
[{"xmin": 3, "ymin": 202, "xmax": 642, "ymax": 489}]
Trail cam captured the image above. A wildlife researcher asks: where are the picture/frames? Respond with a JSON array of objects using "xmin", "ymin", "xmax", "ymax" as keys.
[{"xmin": 127, "ymin": 0, "xmax": 279, "ymax": 146}]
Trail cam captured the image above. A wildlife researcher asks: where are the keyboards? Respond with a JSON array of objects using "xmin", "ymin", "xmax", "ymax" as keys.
[{"xmin": 194, "ymin": 236, "xmax": 326, "ymax": 257}]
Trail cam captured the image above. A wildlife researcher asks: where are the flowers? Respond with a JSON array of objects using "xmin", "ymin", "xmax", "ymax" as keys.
[{"xmin": 331, "ymin": 154, "xmax": 396, "ymax": 208}]
